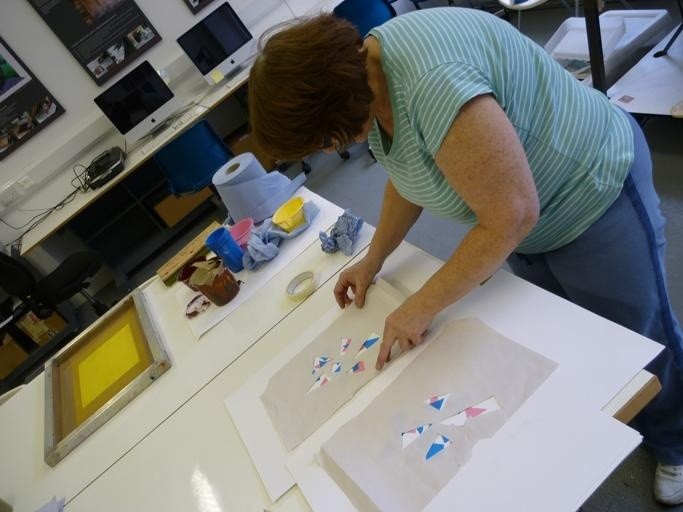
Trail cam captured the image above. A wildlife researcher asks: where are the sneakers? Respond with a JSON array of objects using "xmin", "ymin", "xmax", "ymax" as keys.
[{"xmin": 653, "ymin": 461, "xmax": 682, "ymax": 506}]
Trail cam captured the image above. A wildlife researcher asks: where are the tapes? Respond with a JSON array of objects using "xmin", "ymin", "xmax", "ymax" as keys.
[{"xmin": 287, "ymin": 271, "xmax": 318, "ymax": 303}]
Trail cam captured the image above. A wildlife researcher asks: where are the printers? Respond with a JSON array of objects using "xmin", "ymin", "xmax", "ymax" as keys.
[{"xmin": 86, "ymin": 147, "xmax": 125, "ymax": 191}]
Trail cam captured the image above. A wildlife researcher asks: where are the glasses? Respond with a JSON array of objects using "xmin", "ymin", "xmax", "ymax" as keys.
[{"xmin": 318, "ymin": 133, "xmax": 332, "ymax": 149}]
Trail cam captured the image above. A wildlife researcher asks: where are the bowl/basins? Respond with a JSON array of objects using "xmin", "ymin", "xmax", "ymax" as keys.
[
  {"xmin": 272, "ymin": 196, "xmax": 308, "ymax": 232},
  {"xmin": 229, "ymin": 218, "xmax": 256, "ymax": 248}
]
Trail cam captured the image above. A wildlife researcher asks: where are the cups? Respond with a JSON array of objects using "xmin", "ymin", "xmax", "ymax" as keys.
[{"xmin": 204, "ymin": 227, "xmax": 245, "ymax": 273}]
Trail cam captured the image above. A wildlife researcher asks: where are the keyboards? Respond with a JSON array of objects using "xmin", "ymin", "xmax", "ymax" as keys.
[
  {"xmin": 140, "ymin": 111, "xmax": 193, "ymax": 156},
  {"xmin": 224, "ymin": 63, "xmax": 253, "ymax": 90}
]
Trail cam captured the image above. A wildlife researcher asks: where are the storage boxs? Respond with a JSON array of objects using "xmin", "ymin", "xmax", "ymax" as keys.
[
  {"xmin": 225, "ymin": 123, "xmax": 276, "ymax": 173},
  {"xmin": 17, "ymin": 301, "xmax": 68, "ymax": 347},
  {"xmin": 0, "ymin": 330, "xmax": 27, "ymax": 381},
  {"xmin": 153, "ymin": 186, "xmax": 214, "ymax": 228},
  {"xmin": 544, "ymin": 8, "xmax": 670, "ymax": 129},
  {"xmin": 157, "ymin": 119, "xmax": 233, "ymax": 198}
]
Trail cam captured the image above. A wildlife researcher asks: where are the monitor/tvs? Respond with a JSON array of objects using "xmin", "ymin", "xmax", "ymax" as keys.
[
  {"xmin": 176, "ymin": 1, "xmax": 256, "ymax": 87},
  {"xmin": 94, "ymin": 59, "xmax": 182, "ymax": 146}
]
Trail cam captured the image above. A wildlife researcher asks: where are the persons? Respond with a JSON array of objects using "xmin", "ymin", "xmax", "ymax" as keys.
[{"xmin": 248, "ymin": 6, "xmax": 683, "ymax": 506}]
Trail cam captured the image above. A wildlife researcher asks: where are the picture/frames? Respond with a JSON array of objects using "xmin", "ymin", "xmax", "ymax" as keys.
[
  {"xmin": 44, "ymin": 288, "xmax": 172, "ymax": 466},
  {"xmin": 0, "ymin": 36, "xmax": 66, "ymax": 161},
  {"xmin": 183, "ymin": 0, "xmax": 214, "ymax": 15},
  {"xmin": 27, "ymin": 0, "xmax": 163, "ymax": 87}
]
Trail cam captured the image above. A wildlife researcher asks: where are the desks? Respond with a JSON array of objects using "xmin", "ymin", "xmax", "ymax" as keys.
[
  {"xmin": 19, "ymin": 58, "xmax": 311, "ymax": 315},
  {"xmin": 0, "ymin": 184, "xmax": 662, "ymax": 512}
]
